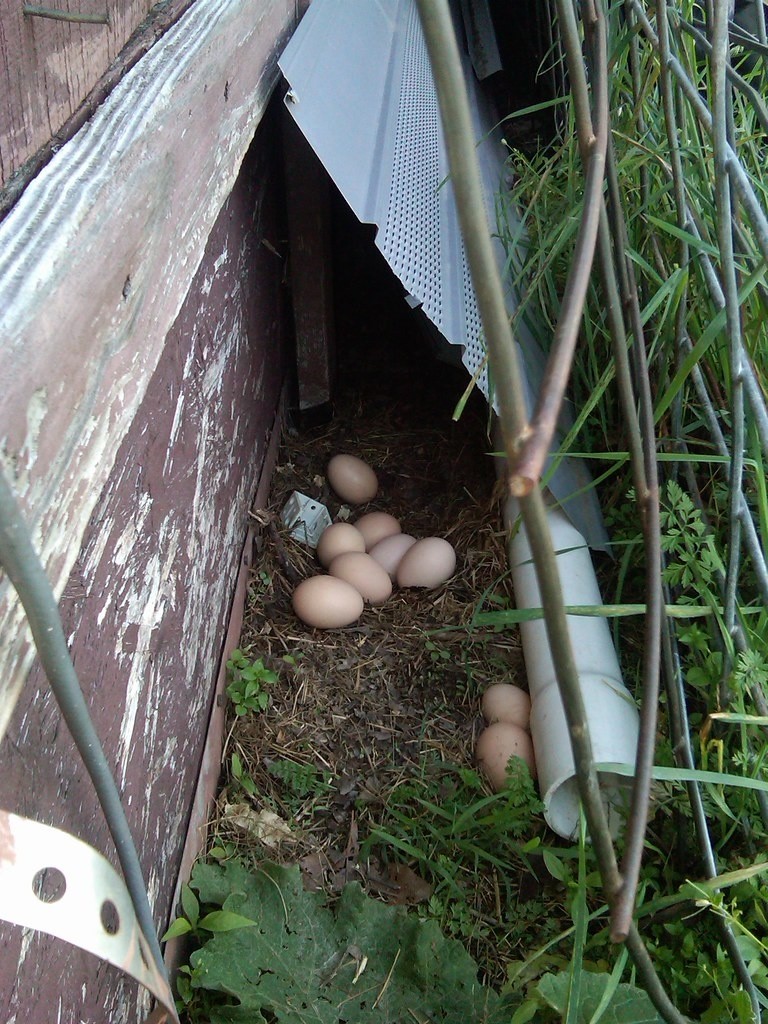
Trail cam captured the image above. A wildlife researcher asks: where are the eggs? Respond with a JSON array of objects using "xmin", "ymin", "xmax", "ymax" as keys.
[
  {"xmin": 477, "ymin": 683, "xmax": 533, "ymax": 793},
  {"xmin": 291, "ymin": 453, "xmax": 457, "ymax": 628}
]
